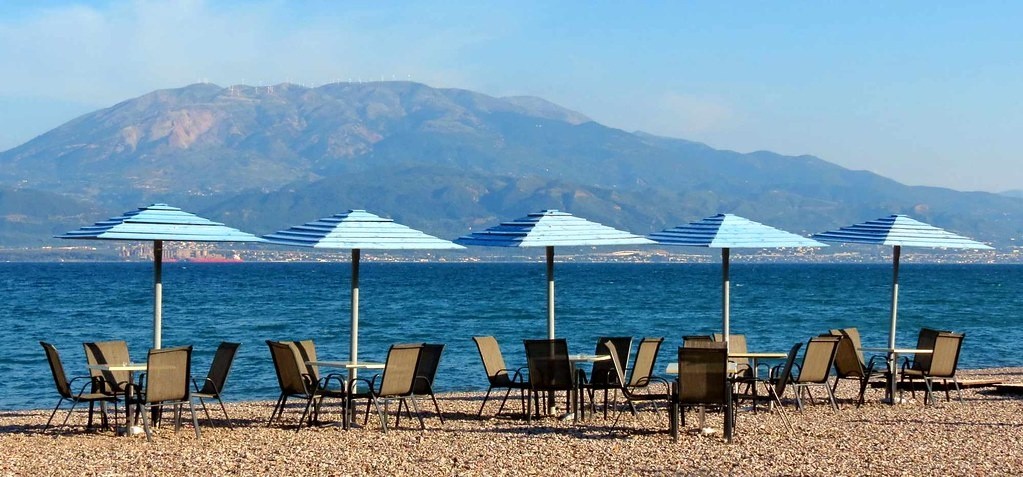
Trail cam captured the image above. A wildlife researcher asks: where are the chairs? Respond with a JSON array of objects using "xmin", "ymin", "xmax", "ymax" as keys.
[
  {"xmin": 123, "ymin": 344, "xmax": 202, "ymax": 443},
  {"xmin": 362, "ymin": 343, "xmax": 447, "ymax": 426},
  {"xmin": 343, "ymin": 342, "xmax": 426, "ymax": 436},
  {"xmin": 157, "ymin": 341, "xmax": 243, "ymax": 432},
  {"xmin": 37, "ymin": 341, "xmax": 122, "ymax": 441},
  {"xmin": 264, "ymin": 339, "xmax": 348, "ymax": 434},
  {"xmin": 469, "ymin": 324, "xmax": 967, "ymax": 446},
  {"xmin": 83, "ymin": 340, "xmax": 148, "ymax": 431},
  {"xmin": 278, "ymin": 339, "xmax": 350, "ymax": 425}
]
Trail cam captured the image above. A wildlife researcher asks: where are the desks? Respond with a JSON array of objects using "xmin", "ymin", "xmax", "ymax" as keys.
[
  {"xmin": 304, "ymin": 360, "xmax": 397, "ymax": 430},
  {"xmin": 524, "ymin": 351, "xmax": 612, "ymax": 421},
  {"xmin": 665, "ymin": 361, "xmax": 739, "ymax": 436},
  {"xmin": 725, "ymin": 352, "xmax": 787, "ymax": 414},
  {"xmin": 857, "ymin": 347, "xmax": 935, "ymax": 406},
  {"xmin": 84, "ymin": 359, "xmax": 176, "ymax": 437}
]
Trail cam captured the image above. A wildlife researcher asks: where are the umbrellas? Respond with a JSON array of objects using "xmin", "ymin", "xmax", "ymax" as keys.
[
  {"xmin": 452, "ymin": 209, "xmax": 659, "ymax": 417},
  {"xmin": 646, "ymin": 213, "xmax": 831, "ymax": 352},
  {"xmin": 261, "ymin": 210, "xmax": 468, "ymax": 394},
  {"xmin": 52, "ymin": 204, "xmax": 260, "ymax": 350},
  {"xmin": 808, "ymin": 213, "xmax": 996, "ymax": 376}
]
